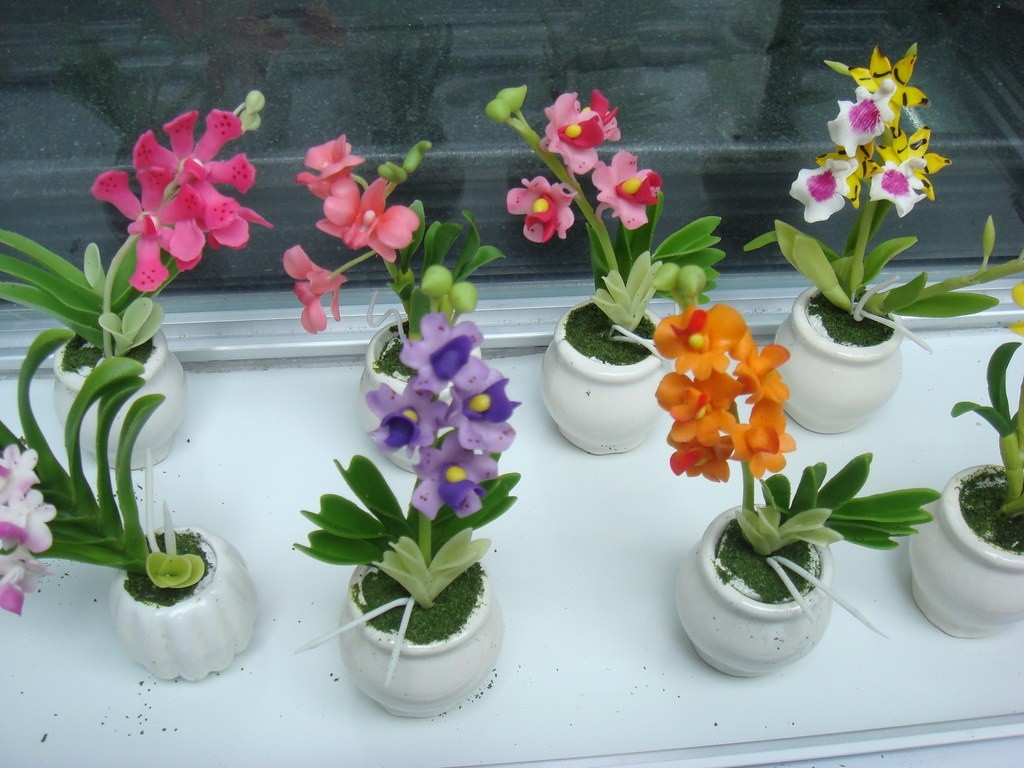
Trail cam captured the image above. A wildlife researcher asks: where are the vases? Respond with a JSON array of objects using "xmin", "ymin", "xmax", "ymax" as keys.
[
  {"xmin": 357, "ymin": 319, "xmax": 482, "ymax": 474},
  {"xmin": 107, "ymin": 525, "xmax": 259, "ymax": 681},
  {"xmin": 673, "ymin": 502, "xmax": 834, "ymax": 678},
  {"xmin": 336, "ymin": 559, "xmax": 503, "ymax": 717},
  {"xmin": 771, "ymin": 284, "xmax": 909, "ymax": 434},
  {"xmin": 52, "ymin": 329, "xmax": 185, "ymax": 471},
  {"xmin": 906, "ymin": 463, "xmax": 1023, "ymax": 640},
  {"xmin": 538, "ymin": 298, "xmax": 668, "ymax": 456}
]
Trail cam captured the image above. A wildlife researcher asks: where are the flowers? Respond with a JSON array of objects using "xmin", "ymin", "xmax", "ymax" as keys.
[
  {"xmin": 949, "ymin": 280, "xmax": 1024, "ymax": 517},
  {"xmin": 484, "ymin": 84, "xmax": 728, "ymax": 363},
  {"xmin": 293, "ymin": 312, "xmax": 523, "ymax": 687},
  {"xmin": 282, "ymin": 133, "xmax": 506, "ymax": 343},
  {"xmin": 0, "ymin": 90, "xmax": 276, "ymax": 360},
  {"xmin": 653, "ymin": 303, "xmax": 944, "ymax": 639},
  {"xmin": 742, "ymin": 42, "xmax": 1024, "ymax": 354},
  {"xmin": 0, "ymin": 326, "xmax": 206, "ymax": 618}
]
